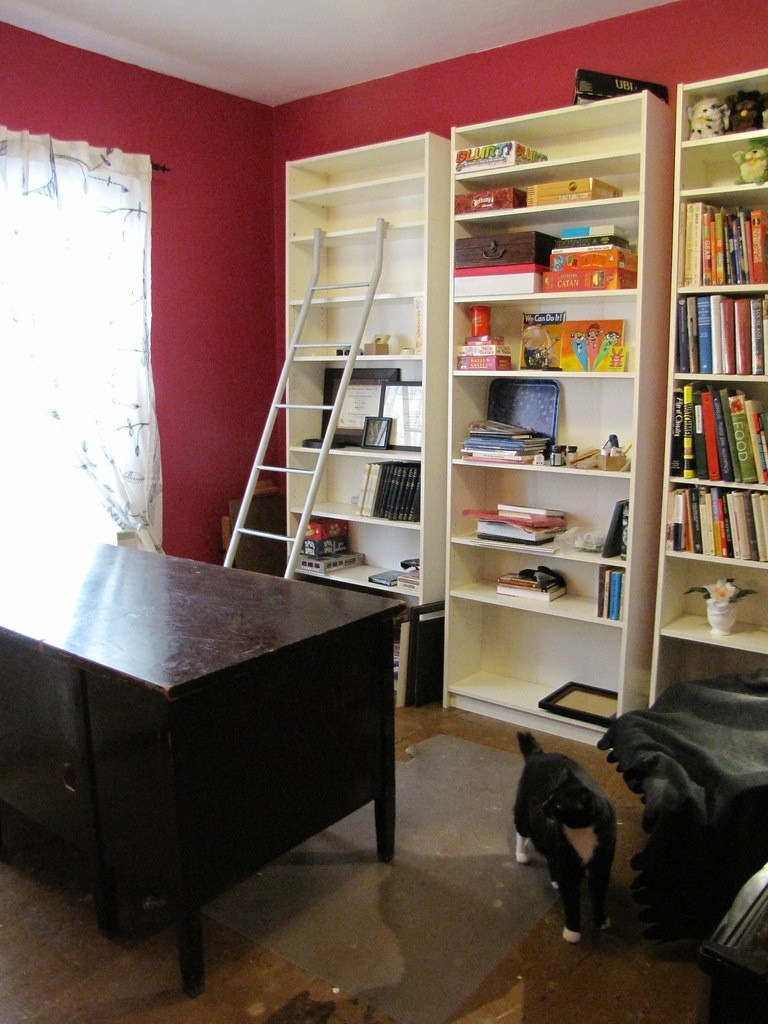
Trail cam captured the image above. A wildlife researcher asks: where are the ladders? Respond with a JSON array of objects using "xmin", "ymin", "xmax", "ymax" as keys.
[{"xmin": 221, "ymin": 218, "xmax": 392, "ymax": 580}]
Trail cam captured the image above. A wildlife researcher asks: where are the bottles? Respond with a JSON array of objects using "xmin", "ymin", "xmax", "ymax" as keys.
[
  {"xmin": 567, "ymin": 446, "xmax": 577, "ymax": 467},
  {"xmin": 550, "ymin": 446, "xmax": 562, "ymax": 465},
  {"xmin": 560, "ymin": 445, "xmax": 566, "ymax": 466}
]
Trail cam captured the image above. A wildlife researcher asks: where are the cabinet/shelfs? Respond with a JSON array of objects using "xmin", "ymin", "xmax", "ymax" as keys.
[
  {"xmin": 443, "ymin": 90, "xmax": 678, "ymax": 751},
  {"xmin": 283, "ymin": 130, "xmax": 450, "ymax": 705},
  {"xmin": 646, "ymin": 65, "xmax": 768, "ymax": 710},
  {"xmin": 1, "ymin": 529, "xmax": 411, "ymax": 1002}
]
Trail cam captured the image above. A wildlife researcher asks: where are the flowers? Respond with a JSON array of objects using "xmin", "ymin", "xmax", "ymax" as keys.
[{"xmin": 681, "ymin": 578, "xmax": 759, "ymax": 607}]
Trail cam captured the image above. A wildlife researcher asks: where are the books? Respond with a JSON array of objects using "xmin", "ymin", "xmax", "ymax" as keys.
[
  {"xmin": 665, "ymin": 203, "xmax": 768, "ymax": 562},
  {"xmin": 461, "ymin": 419, "xmax": 629, "ymax": 621},
  {"xmin": 356, "ymin": 460, "xmax": 421, "ymax": 587}
]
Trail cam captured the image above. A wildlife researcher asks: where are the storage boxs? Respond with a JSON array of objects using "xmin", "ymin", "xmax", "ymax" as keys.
[
  {"xmin": 362, "ymin": 343, "xmax": 390, "ymax": 355},
  {"xmin": 457, "ymin": 336, "xmax": 511, "ymax": 370},
  {"xmin": 453, "ymin": 140, "xmax": 547, "ymax": 175},
  {"xmin": 525, "ymin": 176, "xmax": 623, "ymax": 207},
  {"xmin": 298, "ymin": 538, "xmax": 349, "ymax": 556},
  {"xmin": 597, "ymin": 454, "xmax": 627, "ymax": 470},
  {"xmin": 302, "ymin": 517, "xmax": 349, "ymax": 539},
  {"xmin": 454, "ymin": 185, "xmax": 527, "ymax": 216},
  {"xmin": 454, "ymin": 230, "xmax": 562, "ymax": 266},
  {"xmin": 452, "ymin": 263, "xmax": 549, "ymax": 298},
  {"xmin": 540, "ymin": 224, "xmax": 637, "ymax": 292},
  {"xmin": 296, "ymin": 551, "xmax": 364, "ymax": 573},
  {"xmin": 570, "ymin": 67, "xmax": 668, "ymax": 105}
]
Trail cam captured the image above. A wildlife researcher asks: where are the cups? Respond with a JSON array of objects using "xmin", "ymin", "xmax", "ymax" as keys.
[{"xmin": 472, "ymin": 306, "xmax": 490, "ymax": 336}]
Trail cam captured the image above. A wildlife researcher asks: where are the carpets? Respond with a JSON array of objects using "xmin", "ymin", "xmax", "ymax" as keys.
[{"xmin": 198, "ymin": 733, "xmax": 560, "ymax": 1023}]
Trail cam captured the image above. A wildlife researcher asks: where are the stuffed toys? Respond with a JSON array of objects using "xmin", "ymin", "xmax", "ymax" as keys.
[
  {"xmin": 732, "ymin": 136, "xmax": 768, "ymax": 185},
  {"xmin": 686, "ymin": 90, "xmax": 768, "ymax": 141}
]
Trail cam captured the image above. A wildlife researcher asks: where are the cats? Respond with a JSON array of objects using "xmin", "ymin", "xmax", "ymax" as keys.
[{"xmin": 511, "ymin": 725, "xmax": 619, "ymax": 947}]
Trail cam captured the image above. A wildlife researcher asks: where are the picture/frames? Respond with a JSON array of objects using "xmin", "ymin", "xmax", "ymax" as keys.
[
  {"xmin": 378, "ymin": 380, "xmax": 422, "ymax": 452},
  {"xmin": 361, "ymin": 416, "xmax": 393, "ymax": 451},
  {"xmin": 319, "ymin": 367, "xmax": 401, "ymax": 446},
  {"xmin": 538, "ymin": 679, "xmax": 618, "ymax": 729}
]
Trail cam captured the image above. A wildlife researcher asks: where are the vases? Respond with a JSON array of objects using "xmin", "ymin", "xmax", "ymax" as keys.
[{"xmin": 706, "ymin": 602, "xmax": 737, "ymax": 635}]
